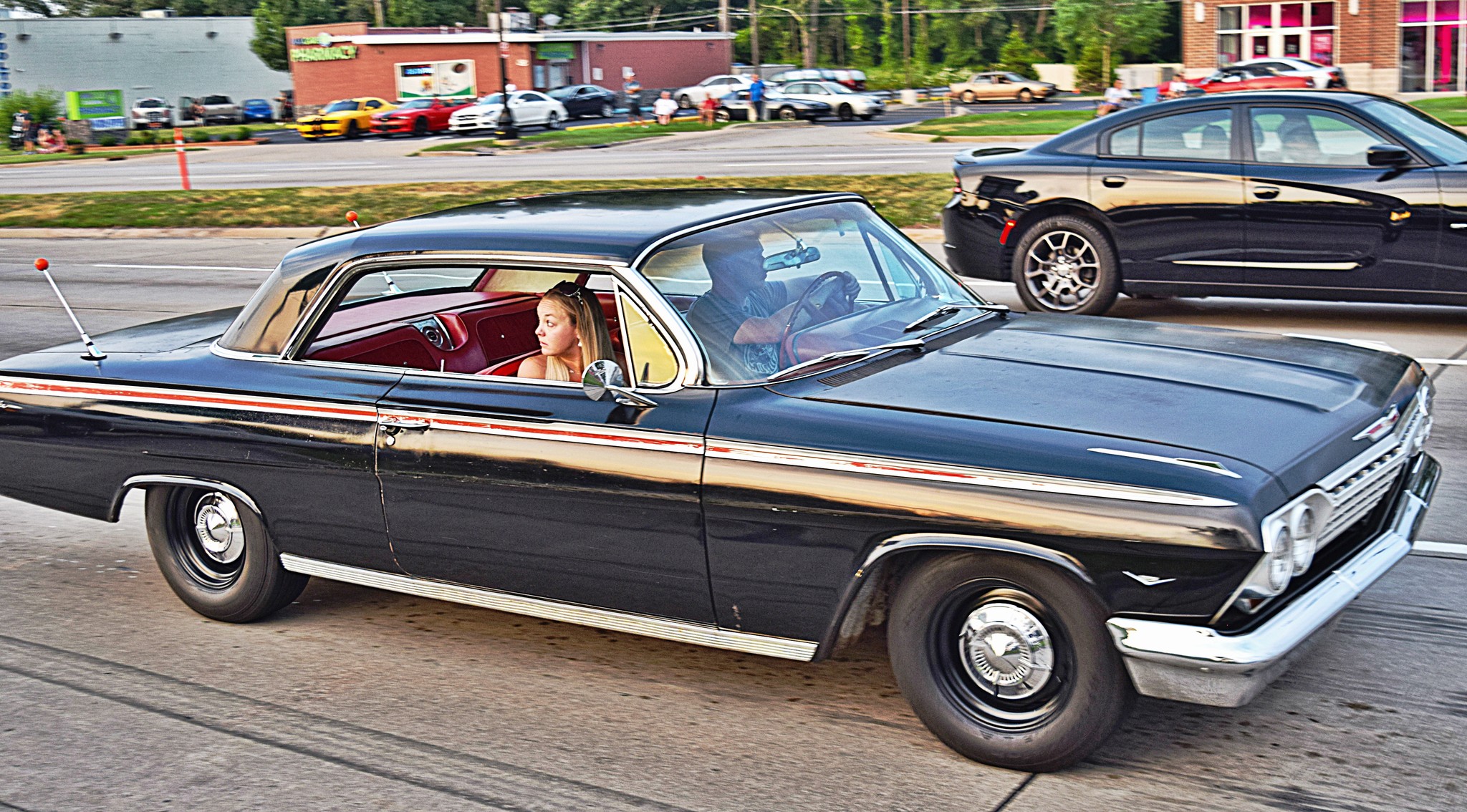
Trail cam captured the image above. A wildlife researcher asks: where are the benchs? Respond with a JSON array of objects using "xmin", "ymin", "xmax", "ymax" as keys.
[{"xmin": 305, "ymin": 292, "xmax": 687, "ymax": 387}]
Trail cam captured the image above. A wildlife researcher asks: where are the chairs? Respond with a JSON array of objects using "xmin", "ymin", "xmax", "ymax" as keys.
[
  {"xmin": 1158, "ymin": 118, "xmax": 1315, "ymax": 163},
  {"xmin": 1092, "ymin": 86, "xmax": 1159, "ymax": 118}
]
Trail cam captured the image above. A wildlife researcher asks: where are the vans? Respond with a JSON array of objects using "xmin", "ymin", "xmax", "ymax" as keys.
[{"xmin": 766, "ymin": 68, "xmax": 867, "ymax": 91}]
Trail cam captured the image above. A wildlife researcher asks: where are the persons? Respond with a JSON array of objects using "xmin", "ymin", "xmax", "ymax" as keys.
[
  {"xmin": 1326, "ymin": 71, "xmax": 1346, "ymax": 89},
  {"xmin": 686, "ymin": 225, "xmax": 861, "ymax": 381},
  {"xmin": 622, "ymin": 73, "xmax": 646, "ymax": 124},
  {"xmin": 1166, "ymin": 74, "xmax": 1188, "ymax": 92},
  {"xmin": 518, "ymin": 280, "xmax": 629, "ymax": 385},
  {"xmin": 193, "ymin": 100, "xmax": 206, "ymax": 126},
  {"xmin": 653, "ymin": 90, "xmax": 679, "ymax": 125},
  {"xmin": 750, "ymin": 75, "xmax": 766, "ymax": 122},
  {"xmin": 275, "ymin": 91, "xmax": 288, "ymax": 119},
  {"xmin": 1102, "ymin": 79, "xmax": 1133, "ymax": 103},
  {"xmin": 702, "ymin": 92, "xmax": 719, "ymax": 128},
  {"xmin": 9, "ymin": 107, "xmax": 68, "ymax": 154},
  {"xmin": 1277, "ymin": 118, "xmax": 1367, "ymax": 166}
]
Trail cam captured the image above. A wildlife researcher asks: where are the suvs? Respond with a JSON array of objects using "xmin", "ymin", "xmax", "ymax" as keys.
[{"xmin": 130, "ymin": 96, "xmax": 175, "ymax": 130}]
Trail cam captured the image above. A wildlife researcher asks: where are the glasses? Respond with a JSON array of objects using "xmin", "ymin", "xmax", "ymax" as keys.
[{"xmin": 552, "ymin": 280, "xmax": 584, "ymax": 305}]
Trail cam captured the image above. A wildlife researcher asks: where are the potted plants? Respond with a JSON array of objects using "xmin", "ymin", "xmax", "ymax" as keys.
[{"xmin": 66, "ymin": 139, "xmax": 84, "ymax": 155}]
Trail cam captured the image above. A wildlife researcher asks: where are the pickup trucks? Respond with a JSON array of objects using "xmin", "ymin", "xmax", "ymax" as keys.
[{"xmin": 177, "ymin": 95, "xmax": 237, "ymax": 127}]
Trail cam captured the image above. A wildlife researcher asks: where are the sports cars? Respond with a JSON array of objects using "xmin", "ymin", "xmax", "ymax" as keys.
[
  {"xmin": 296, "ymin": 97, "xmax": 400, "ymax": 142},
  {"xmin": 367, "ymin": 98, "xmax": 475, "ymax": 139}
]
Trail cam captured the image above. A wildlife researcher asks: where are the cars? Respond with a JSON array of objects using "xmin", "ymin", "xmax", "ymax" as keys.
[
  {"xmin": 703, "ymin": 88, "xmax": 832, "ymax": 121},
  {"xmin": 447, "ymin": 89, "xmax": 568, "ymax": 137},
  {"xmin": 543, "ymin": 84, "xmax": 620, "ymax": 118},
  {"xmin": 672, "ymin": 74, "xmax": 778, "ymax": 113},
  {"xmin": 1156, "ymin": 66, "xmax": 1315, "ymax": 106},
  {"xmin": 773, "ymin": 81, "xmax": 887, "ymax": 122},
  {"xmin": 1223, "ymin": 58, "xmax": 1349, "ymax": 88},
  {"xmin": 237, "ymin": 99, "xmax": 273, "ymax": 125},
  {"xmin": 1, "ymin": 187, "xmax": 1441, "ymax": 775},
  {"xmin": 940, "ymin": 88, "xmax": 1467, "ymax": 316},
  {"xmin": 946, "ymin": 71, "xmax": 1057, "ymax": 104}
]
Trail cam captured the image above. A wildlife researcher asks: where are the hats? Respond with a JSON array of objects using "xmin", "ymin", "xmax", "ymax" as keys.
[{"xmin": 625, "ymin": 72, "xmax": 635, "ymax": 77}]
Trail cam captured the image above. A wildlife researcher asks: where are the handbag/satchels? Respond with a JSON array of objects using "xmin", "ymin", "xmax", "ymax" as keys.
[{"xmin": 747, "ymin": 103, "xmax": 757, "ymax": 122}]
